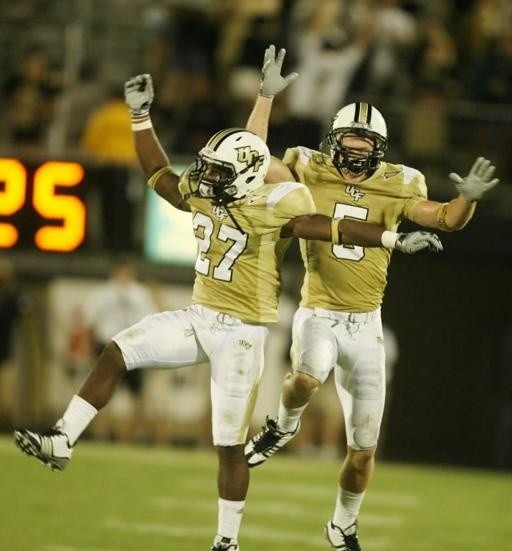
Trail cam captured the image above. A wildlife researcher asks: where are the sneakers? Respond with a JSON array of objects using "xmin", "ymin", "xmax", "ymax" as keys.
[
  {"xmin": 210, "ymin": 534, "xmax": 238, "ymax": 551},
  {"xmin": 12, "ymin": 419, "xmax": 78, "ymax": 473},
  {"xmin": 245, "ymin": 417, "xmax": 301, "ymax": 467},
  {"xmin": 323, "ymin": 515, "xmax": 361, "ymax": 550}
]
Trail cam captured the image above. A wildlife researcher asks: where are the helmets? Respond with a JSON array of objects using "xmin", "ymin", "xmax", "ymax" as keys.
[
  {"xmin": 320, "ymin": 102, "xmax": 388, "ymax": 181},
  {"xmin": 188, "ymin": 127, "xmax": 271, "ymax": 206}
]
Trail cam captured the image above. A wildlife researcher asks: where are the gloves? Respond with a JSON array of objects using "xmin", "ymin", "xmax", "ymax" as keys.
[
  {"xmin": 259, "ymin": 45, "xmax": 297, "ymax": 98},
  {"xmin": 395, "ymin": 230, "xmax": 443, "ymax": 255},
  {"xmin": 124, "ymin": 73, "xmax": 155, "ymax": 116},
  {"xmin": 450, "ymin": 156, "xmax": 499, "ymax": 203}
]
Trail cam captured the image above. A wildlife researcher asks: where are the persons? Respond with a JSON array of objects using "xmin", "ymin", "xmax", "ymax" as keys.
[
  {"xmin": 1, "ymin": 1, "xmax": 512, "ymax": 452},
  {"xmin": 243, "ymin": 43, "xmax": 500, "ymax": 550},
  {"xmin": 12, "ymin": 73, "xmax": 446, "ymax": 551}
]
